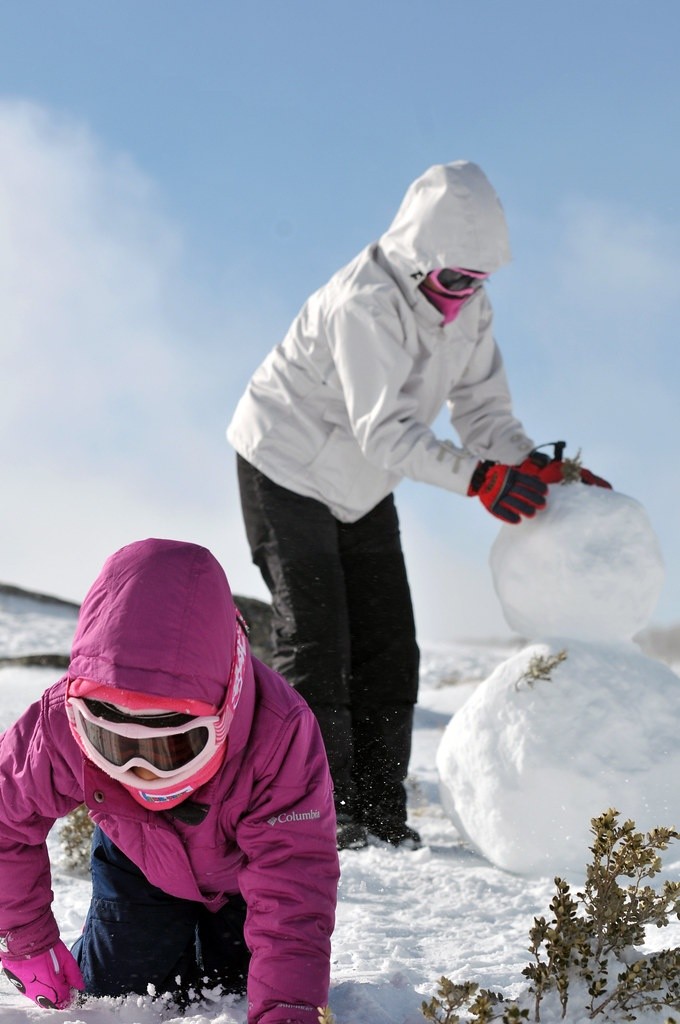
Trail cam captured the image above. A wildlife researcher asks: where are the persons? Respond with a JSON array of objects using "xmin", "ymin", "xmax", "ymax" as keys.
[
  {"xmin": 1, "ymin": 539, "xmax": 340, "ymax": 1024},
  {"xmin": 219, "ymin": 156, "xmax": 615, "ymax": 855}
]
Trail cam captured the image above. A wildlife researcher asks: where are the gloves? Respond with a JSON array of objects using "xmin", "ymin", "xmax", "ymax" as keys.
[
  {"xmin": 0, "ymin": 940, "xmax": 84, "ymax": 1010},
  {"xmin": 511, "ymin": 440, "xmax": 613, "ymax": 490},
  {"xmin": 468, "ymin": 459, "xmax": 549, "ymax": 524}
]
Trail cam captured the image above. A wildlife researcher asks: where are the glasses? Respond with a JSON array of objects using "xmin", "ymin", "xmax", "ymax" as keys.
[
  {"xmin": 428, "ymin": 267, "xmax": 488, "ymax": 297},
  {"xmin": 65, "ymin": 606, "xmax": 251, "ymax": 778}
]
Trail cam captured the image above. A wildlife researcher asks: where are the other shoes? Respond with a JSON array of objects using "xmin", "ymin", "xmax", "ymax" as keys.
[{"xmin": 335, "ymin": 813, "xmax": 422, "ymax": 853}]
